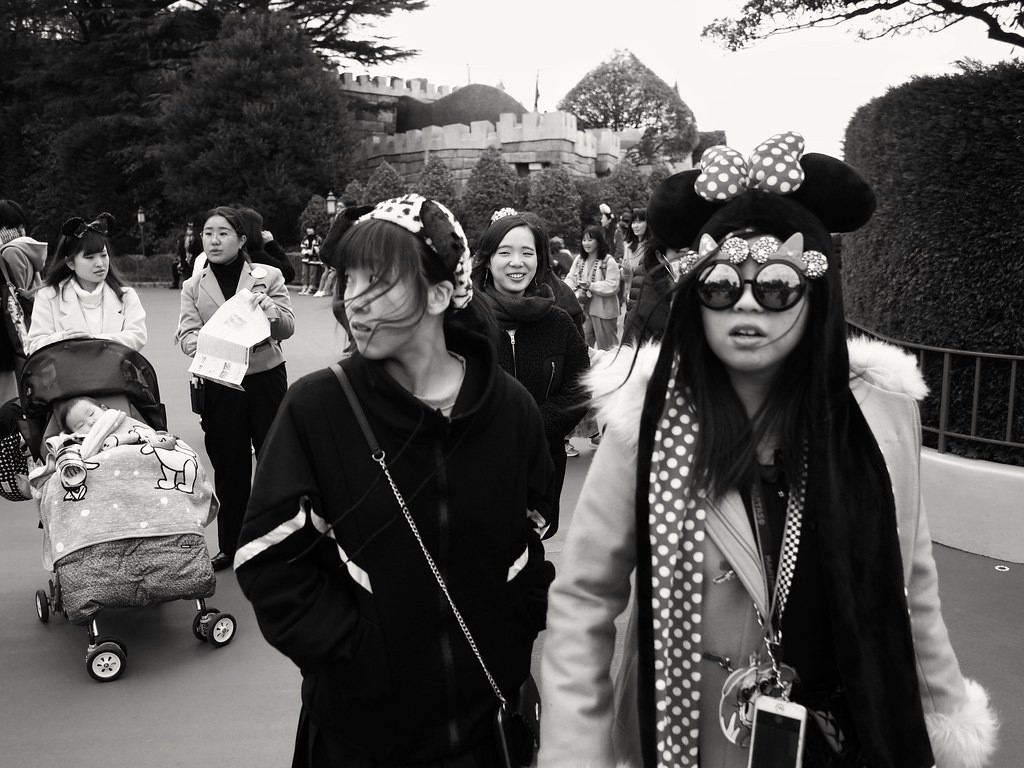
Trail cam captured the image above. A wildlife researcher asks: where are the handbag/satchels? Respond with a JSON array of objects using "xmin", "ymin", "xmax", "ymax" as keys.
[
  {"xmin": 573, "ymin": 286, "xmax": 593, "ymax": 305},
  {"xmin": 0, "ymin": 392, "xmax": 36, "ymax": 502},
  {"xmin": 189, "ymin": 376, "xmax": 205, "ymax": 414},
  {"xmin": 493, "ymin": 675, "xmax": 540, "ymax": 768},
  {"xmin": 0, "ymin": 254, "xmax": 33, "ymax": 373}
]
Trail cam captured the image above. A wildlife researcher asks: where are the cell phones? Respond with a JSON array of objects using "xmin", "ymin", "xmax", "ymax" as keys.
[{"xmin": 747, "ymin": 695, "xmax": 807, "ymax": 768}]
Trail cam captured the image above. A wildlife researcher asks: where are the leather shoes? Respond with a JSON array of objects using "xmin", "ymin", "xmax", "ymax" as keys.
[{"xmin": 211, "ymin": 550, "xmax": 235, "ymax": 571}]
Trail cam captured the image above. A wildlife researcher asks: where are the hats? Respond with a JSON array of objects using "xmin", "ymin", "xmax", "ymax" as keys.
[{"xmin": 319, "ymin": 193, "xmax": 473, "ymax": 319}]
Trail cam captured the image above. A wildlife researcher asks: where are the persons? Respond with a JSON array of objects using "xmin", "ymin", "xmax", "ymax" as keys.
[
  {"xmin": 55, "ymin": 396, "xmax": 108, "ymax": 486},
  {"xmin": 466, "ymin": 211, "xmax": 591, "ymax": 542},
  {"xmin": 548, "ymin": 205, "xmax": 676, "ymax": 455},
  {"xmin": 26, "ymin": 229, "xmax": 147, "ymax": 353},
  {"xmin": 0, "ymin": 199, "xmax": 49, "ymax": 409},
  {"xmin": 537, "ymin": 190, "xmax": 996, "ymax": 768},
  {"xmin": 168, "ymin": 203, "xmax": 295, "ymax": 569},
  {"xmin": 298, "ymin": 224, "xmax": 337, "ymax": 298},
  {"xmin": 232, "ymin": 191, "xmax": 557, "ymax": 768}
]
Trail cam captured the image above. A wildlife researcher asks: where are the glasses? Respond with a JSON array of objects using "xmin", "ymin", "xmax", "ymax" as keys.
[
  {"xmin": 200, "ymin": 230, "xmax": 237, "ymax": 239},
  {"xmin": 694, "ymin": 258, "xmax": 810, "ymax": 313}
]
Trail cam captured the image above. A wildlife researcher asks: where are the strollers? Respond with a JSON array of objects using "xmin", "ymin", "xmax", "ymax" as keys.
[{"xmin": 17, "ymin": 333, "xmax": 235, "ymax": 681}]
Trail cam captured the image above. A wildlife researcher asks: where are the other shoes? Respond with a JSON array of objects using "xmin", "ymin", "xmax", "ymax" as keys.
[
  {"xmin": 170, "ymin": 284, "xmax": 180, "ymax": 289},
  {"xmin": 590, "ymin": 432, "xmax": 601, "ymax": 448},
  {"xmin": 298, "ymin": 286, "xmax": 334, "ymax": 298},
  {"xmin": 564, "ymin": 442, "xmax": 580, "ymax": 456}
]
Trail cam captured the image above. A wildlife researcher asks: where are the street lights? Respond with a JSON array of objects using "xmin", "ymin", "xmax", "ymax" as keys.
[
  {"xmin": 326, "ymin": 191, "xmax": 337, "ymax": 230},
  {"xmin": 137, "ymin": 206, "xmax": 147, "ymax": 255}
]
